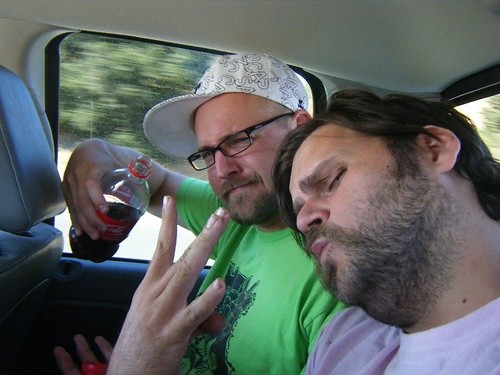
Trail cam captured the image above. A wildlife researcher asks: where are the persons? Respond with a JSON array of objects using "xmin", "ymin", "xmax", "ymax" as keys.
[
  {"xmin": 104, "ymin": 90, "xmax": 499, "ymax": 374},
  {"xmin": 54, "ymin": 53, "xmax": 351, "ymax": 375}
]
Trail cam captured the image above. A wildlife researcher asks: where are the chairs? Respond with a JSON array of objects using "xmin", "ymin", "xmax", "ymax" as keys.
[{"xmin": 0, "ymin": 65, "xmax": 67, "ymax": 375}]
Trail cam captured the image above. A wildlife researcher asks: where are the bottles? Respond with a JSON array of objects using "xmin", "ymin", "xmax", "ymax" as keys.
[{"xmin": 69, "ymin": 155, "xmax": 156, "ymax": 264}]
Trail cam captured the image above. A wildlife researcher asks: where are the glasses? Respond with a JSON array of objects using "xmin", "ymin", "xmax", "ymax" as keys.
[{"xmin": 186, "ymin": 112, "xmax": 295, "ymax": 171}]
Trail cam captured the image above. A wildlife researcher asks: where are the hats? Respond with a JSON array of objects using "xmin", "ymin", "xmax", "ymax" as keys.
[{"xmin": 142, "ymin": 49, "xmax": 310, "ymax": 158}]
